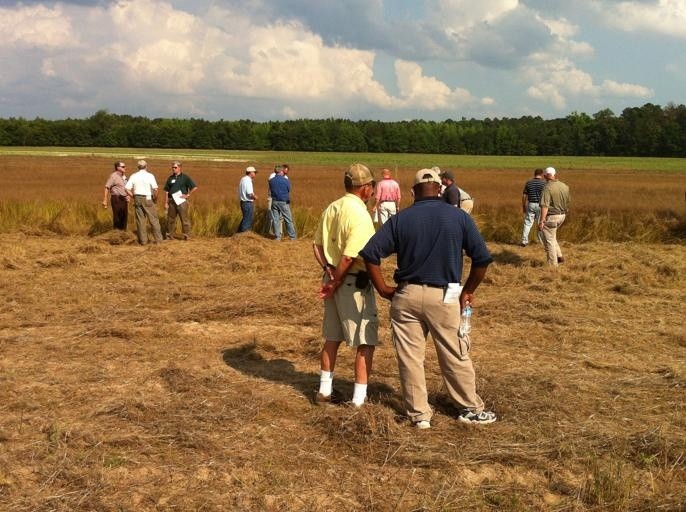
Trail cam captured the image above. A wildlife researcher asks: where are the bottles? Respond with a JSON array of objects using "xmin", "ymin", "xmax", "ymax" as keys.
[{"xmin": 462, "ymin": 301, "xmax": 472, "ymax": 334}]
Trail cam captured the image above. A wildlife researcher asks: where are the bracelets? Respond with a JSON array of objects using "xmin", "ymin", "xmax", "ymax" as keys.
[
  {"xmin": 322, "ymin": 263, "xmax": 330, "ymax": 270},
  {"xmin": 187, "ymin": 194, "xmax": 190, "ymax": 197}
]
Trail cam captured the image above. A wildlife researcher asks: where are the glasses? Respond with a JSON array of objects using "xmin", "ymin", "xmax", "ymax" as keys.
[
  {"xmin": 172, "ymin": 167, "xmax": 177, "ymax": 169},
  {"xmin": 119, "ymin": 166, "xmax": 125, "ymax": 168}
]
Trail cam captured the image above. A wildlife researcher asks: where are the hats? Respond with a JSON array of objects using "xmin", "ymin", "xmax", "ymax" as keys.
[
  {"xmin": 544, "ymin": 167, "xmax": 555, "ymax": 175},
  {"xmin": 383, "ymin": 169, "xmax": 390, "ymax": 178},
  {"xmin": 246, "ymin": 167, "xmax": 257, "ymax": 172},
  {"xmin": 137, "ymin": 160, "xmax": 147, "ymax": 167},
  {"xmin": 345, "ymin": 162, "xmax": 376, "ymax": 186},
  {"xmin": 413, "ymin": 168, "xmax": 454, "ymax": 185}
]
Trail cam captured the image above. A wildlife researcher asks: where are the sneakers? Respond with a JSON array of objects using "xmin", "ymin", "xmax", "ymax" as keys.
[
  {"xmin": 314, "ymin": 391, "xmax": 351, "ymax": 405},
  {"xmin": 458, "ymin": 410, "xmax": 497, "ymax": 424},
  {"xmin": 559, "ymin": 257, "xmax": 564, "ymax": 263},
  {"xmin": 414, "ymin": 420, "xmax": 431, "ymax": 429}
]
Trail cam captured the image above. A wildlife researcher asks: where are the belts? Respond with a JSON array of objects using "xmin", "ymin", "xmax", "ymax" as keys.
[{"xmin": 408, "ymin": 280, "xmax": 440, "ymax": 286}]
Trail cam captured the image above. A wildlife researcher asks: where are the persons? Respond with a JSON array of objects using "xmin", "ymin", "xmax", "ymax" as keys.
[
  {"xmin": 439, "ymin": 171, "xmax": 460, "ymax": 208},
  {"xmin": 372, "ymin": 169, "xmax": 402, "ymax": 225},
  {"xmin": 124, "ymin": 160, "xmax": 163, "ymax": 245},
  {"xmin": 239, "ymin": 165, "xmax": 297, "ymax": 241},
  {"xmin": 520, "ymin": 169, "xmax": 547, "ymax": 247},
  {"xmin": 311, "ymin": 162, "xmax": 377, "ymax": 413},
  {"xmin": 163, "ymin": 161, "xmax": 197, "ymax": 240},
  {"xmin": 538, "ymin": 166, "xmax": 571, "ymax": 267},
  {"xmin": 360, "ymin": 168, "xmax": 497, "ymax": 430},
  {"xmin": 102, "ymin": 161, "xmax": 129, "ymax": 231},
  {"xmin": 431, "ymin": 166, "xmax": 474, "ymax": 213}
]
